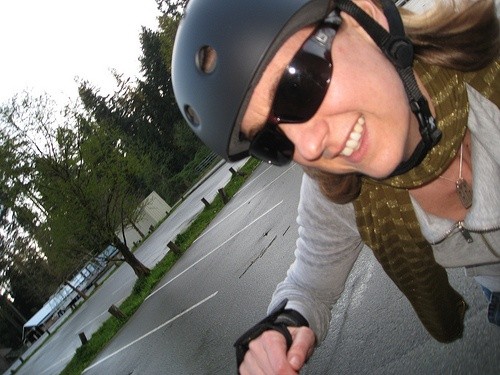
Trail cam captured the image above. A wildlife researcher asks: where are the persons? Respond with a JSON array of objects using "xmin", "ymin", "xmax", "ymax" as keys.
[{"xmin": 171, "ymin": 0, "xmax": 500, "ymax": 375}]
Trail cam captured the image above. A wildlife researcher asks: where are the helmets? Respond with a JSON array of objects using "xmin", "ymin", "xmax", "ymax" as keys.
[{"xmin": 171, "ymin": 0, "xmax": 338, "ymax": 164}]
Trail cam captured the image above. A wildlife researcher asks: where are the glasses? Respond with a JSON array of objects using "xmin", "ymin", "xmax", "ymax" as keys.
[{"xmin": 247, "ymin": 12, "xmax": 342, "ymax": 167}]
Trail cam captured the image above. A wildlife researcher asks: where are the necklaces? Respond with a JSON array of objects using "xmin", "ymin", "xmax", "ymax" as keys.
[{"xmin": 438, "ymin": 141, "xmax": 473, "ymax": 209}]
